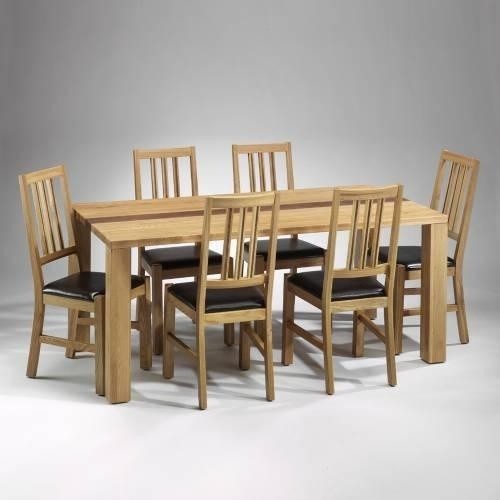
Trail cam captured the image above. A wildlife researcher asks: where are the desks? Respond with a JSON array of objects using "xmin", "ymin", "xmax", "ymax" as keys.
[{"xmin": 69, "ymin": 186, "xmax": 451, "ymax": 404}]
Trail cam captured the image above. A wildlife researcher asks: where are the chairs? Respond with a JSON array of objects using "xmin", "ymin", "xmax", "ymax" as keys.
[
  {"xmin": 130, "ymin": 144, "xmax": 238, "ymax": 358},
  {"xmin": 281, "ymin": 183, "xmax": 407, "ymax": 397},
  {"xmin": 229, "ymin": 141, "xmax": 331, "ymax": 332},
  {"xmin": 17, "ymin": 162, "xmax": 153, "ymax": 398},
  {"xmin": 164, "ymin": 189, "xmax": 284, "ymax": 412},
  {"xmin": 363, "ymin": 146, "xmax": 482, "ymax": 356}
]
